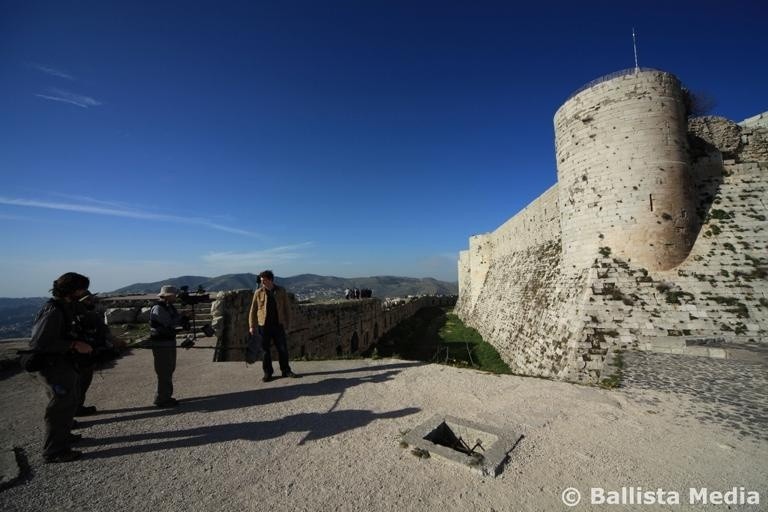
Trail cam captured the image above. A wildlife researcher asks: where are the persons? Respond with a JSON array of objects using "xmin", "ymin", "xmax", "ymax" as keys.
[
  {"xmin": 343, "ymin": 287, "xmax": 373, "ymax": 300},
  {"xmin": 70, "ymin": 290, "xmax": 98, "ymax": 417},
  {"xmin": 29, "ymin": 273, "xmax": 93, "ymax": 463},
  {"xmin": 249, "ymin": 270, "xmax": 297, "ymax": 382},
  {"xmin": 149, "ymin": 285, "xmax": 191, "ymax": 407}
]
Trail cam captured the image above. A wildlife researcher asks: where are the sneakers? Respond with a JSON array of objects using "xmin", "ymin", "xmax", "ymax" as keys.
[
  {"xmin": 261, "ymin": 374, "xmax": 273, "ymax": 383},
  {"xmin": 43, "ymin": 434, "xmax": 81, "ymax": 463},
  {"xmin": 151, "ymin": 398, "xmax": 175, "ymax": 405},
  {"xmin": 282, "ymin": 371, "xmax": 302, "ymax": 379},
  {"xmin": 76, "ymin": 406, "xmax": 97, "ymax": 416}
]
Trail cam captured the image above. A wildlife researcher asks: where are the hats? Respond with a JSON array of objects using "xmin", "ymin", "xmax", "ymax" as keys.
[
  {"xmin": 157, "ymin": 286, "xmax": 177, "ymax": 297},
  {"xmin": 78, "ymin": 290, "xmax": 98, "ymax": 302}
]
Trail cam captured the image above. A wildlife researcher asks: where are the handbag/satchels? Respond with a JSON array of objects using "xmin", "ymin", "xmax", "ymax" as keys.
[{"xmin": 15, "ymin": 345, "xmax": 43, "ymax": 373}]
